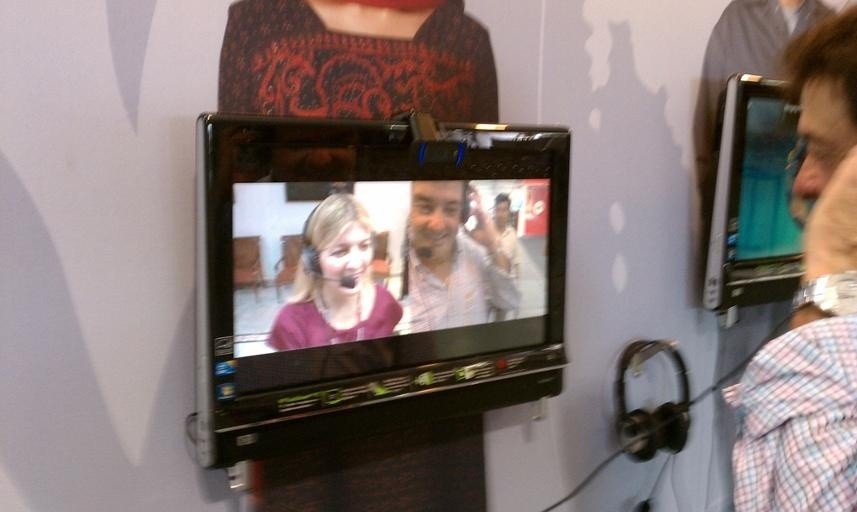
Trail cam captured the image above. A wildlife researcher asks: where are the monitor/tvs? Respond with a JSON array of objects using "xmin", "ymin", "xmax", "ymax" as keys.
[
  {"xmin": 193, "ymin": 110, "xmax": 572, "ymax": 469},
  {"xmin": 701, "ymin": 71, "xmax": 817, "ymax": 312}
]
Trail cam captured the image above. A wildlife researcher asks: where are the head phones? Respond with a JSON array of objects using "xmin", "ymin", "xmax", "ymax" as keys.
[
  {"xmin": 300, "ymin": 200, "xmax": 381, "ymax": 277},
  {"xmin": 613, "ymin": 339, "xmax": 691, "ymax": 463},
  {"xmin": 460, "ymin": 180, "xmax": 473, "ymax": 225}
]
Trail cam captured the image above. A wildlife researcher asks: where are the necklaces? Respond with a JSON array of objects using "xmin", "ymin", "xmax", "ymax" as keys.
[{"xmin": 311, "ymin": 288, "xmax": 366, "ymax": 344}]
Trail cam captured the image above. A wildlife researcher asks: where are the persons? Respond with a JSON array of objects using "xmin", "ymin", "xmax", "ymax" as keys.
[
  {"xmin": 217, "ymin": 1, "xmax": 501, "ymax": 507},
  {"xmin": 717, "ymin": 5, "xmax": 856, "ymax": 512},
  {"xmin": 263, "ymin": 192, "xmax": 404, "ymax": 352},
  {"xmin": 691, "ymin": 0, "xmax": 839, "ymax": 510},
  {"xmin": 401, "ymin": 179, "xmax": 521, "ymax": 335},
  {"xmin": 489, "ymin": 184, "xmax": 527, "ymax": 324}
]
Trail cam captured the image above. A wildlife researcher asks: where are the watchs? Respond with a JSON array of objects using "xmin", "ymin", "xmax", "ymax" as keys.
[{"xmin": 785, "ymin": 270, "xmax": 856, "ymax": 320}]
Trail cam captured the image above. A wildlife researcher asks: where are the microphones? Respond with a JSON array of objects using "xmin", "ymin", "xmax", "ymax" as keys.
[
  {"xmin": 314, "ymin": 277, "xmax": 355, "ymax": 288},
  {"xmin": 633, "ymin": 453, "xmax": 672, "ymax": 512},
  {"xmin": 413, "ymin": 246, "xmax": 433, "ymax": 258}
]
